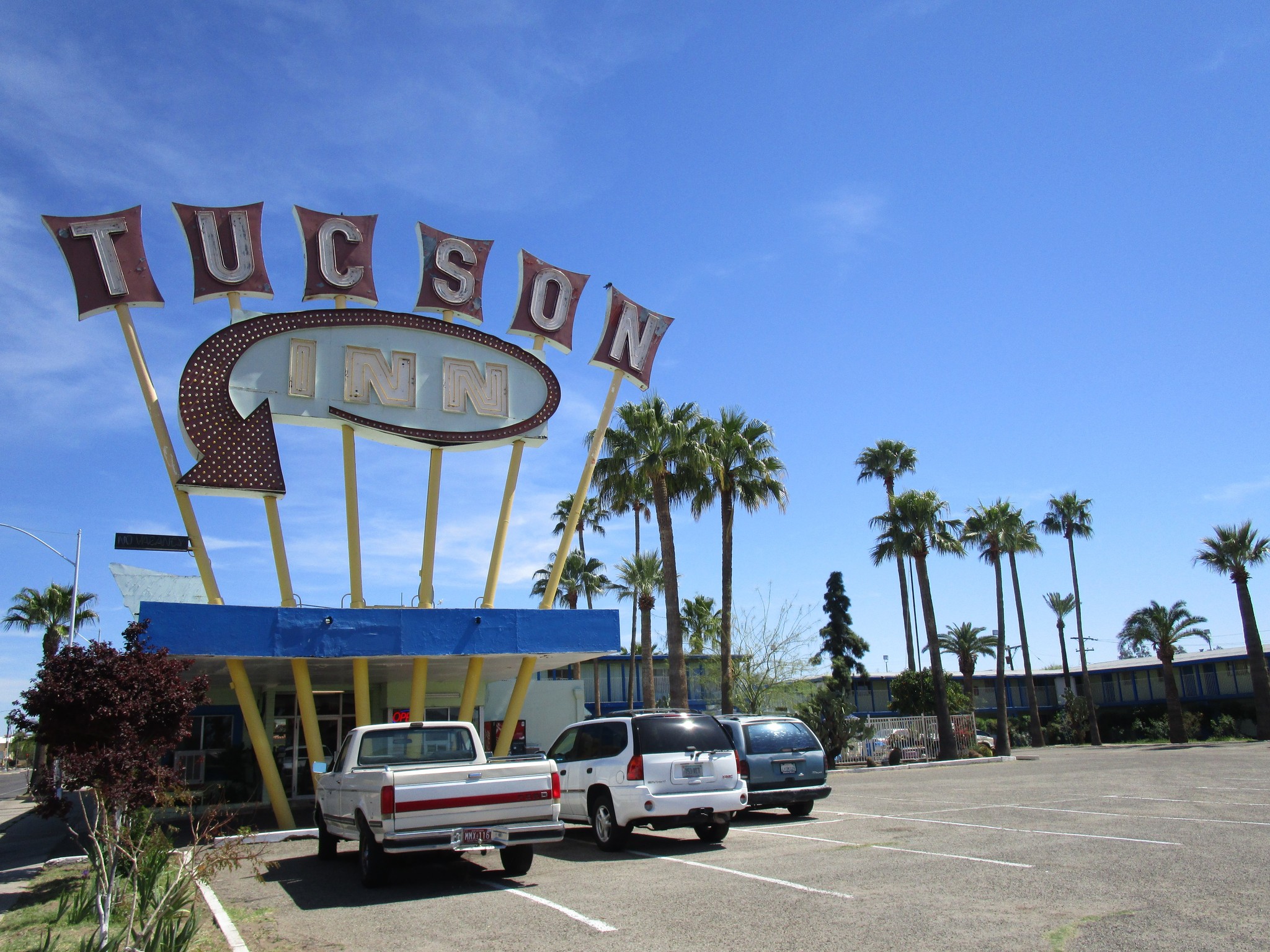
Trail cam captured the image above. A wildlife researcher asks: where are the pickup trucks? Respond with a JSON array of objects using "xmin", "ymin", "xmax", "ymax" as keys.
[
  {"xmin": 281, "ymin": 743, "xmax": 334, "ymax": 771},
  {"xmin": 312, "ymin": 720, "xmax": 566, "ymax": 884}
]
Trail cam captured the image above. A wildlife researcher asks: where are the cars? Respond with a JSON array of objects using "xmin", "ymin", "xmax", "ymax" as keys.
[{"xmin": 839, "ymin": 722, "xmax": 995, "ymax": 761}]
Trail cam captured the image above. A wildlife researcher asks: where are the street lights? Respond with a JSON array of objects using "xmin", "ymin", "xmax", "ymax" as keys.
[
  {"xmin": 1204, "ymin": 629, "xmax": 1211, "ymax": 650},
  {"xmin": 0, "ymin": 710, "xmax": 22, "ymax": 770},
  {"xmin": 883, "ymin": 655, "xmax": 889, "ymax": 672}
]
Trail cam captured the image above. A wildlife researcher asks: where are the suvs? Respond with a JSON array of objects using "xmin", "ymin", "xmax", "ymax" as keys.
[
  {"xmin": 713, "ymin": 714, "xmax": 832, "ymax": 821},
  {"xmin": 533, "ymin": 707, "xmax": 749, "ymax": 853}
]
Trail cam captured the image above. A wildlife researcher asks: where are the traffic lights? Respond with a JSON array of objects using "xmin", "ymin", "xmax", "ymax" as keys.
[{"xmin": 26, "ymin": 753, "xmax": 29, "ymax": 758}]
